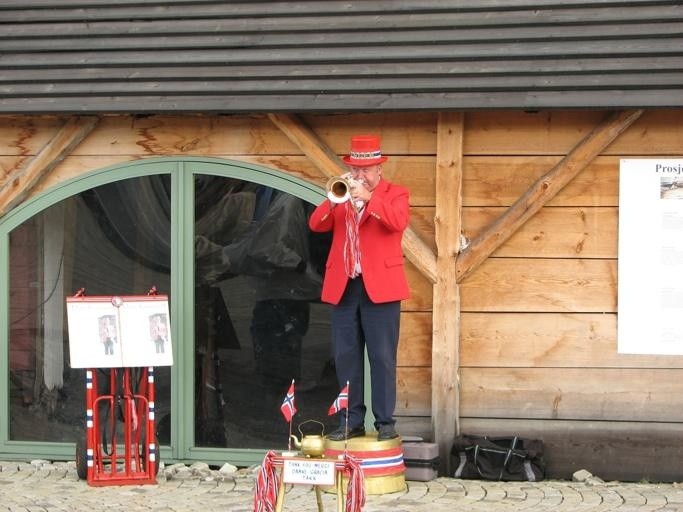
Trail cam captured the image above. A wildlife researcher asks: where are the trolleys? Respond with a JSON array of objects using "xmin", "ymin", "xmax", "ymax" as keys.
[{"xmin": 65, "ymin": 285, "xmax": 162, "ymax": 487}]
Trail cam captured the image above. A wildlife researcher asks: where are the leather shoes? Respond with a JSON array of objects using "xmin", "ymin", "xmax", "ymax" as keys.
[
  {"xmin": 328, "ymin": 425, "xmax": 365, "ymax": 440},
  {"xmin": 377, "ymin": 423, "xmax": 399, "ymax": 441}
]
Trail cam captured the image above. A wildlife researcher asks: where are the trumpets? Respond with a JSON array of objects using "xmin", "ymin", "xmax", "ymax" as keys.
[{"xmin": 326, "ymin": 174, "xmax": 365, "ymax": 213}]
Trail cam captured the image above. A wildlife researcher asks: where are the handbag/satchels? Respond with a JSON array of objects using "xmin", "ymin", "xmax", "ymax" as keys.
[{"xmin": 451, "ymin": 434, "xmax": 546, "ymax": 482}]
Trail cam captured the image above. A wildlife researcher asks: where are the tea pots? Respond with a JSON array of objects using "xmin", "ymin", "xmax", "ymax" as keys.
[{"xmin": 290, "ymin": 419, "xmax": 327, "ymax": 458}]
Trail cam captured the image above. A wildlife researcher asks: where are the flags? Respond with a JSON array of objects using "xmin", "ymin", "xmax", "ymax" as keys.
[
  {"xmin": 327, "ymin": 386, "xmax": 349, "ymax": 416},
  {"xmin": 280, "ymin": 383, "xmax": 298, "ymax": 423}
]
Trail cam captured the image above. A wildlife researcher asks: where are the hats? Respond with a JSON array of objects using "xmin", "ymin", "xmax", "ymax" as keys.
[{"xmin": 344, "ymin": 135, "xmax": 388, "ymax": 167}]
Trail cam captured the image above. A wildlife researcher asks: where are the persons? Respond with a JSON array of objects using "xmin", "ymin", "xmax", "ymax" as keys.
[
  {"xmin": 150, "ymin": 316, "xmax": 168, "ymax": 353},
  {"xmin": 307, "ymin": 134, "xmax": 413, "ymax": 439},
  {"xmin": 101, "ymin": 315, "xmax": 116, "ymax": 356}
]
